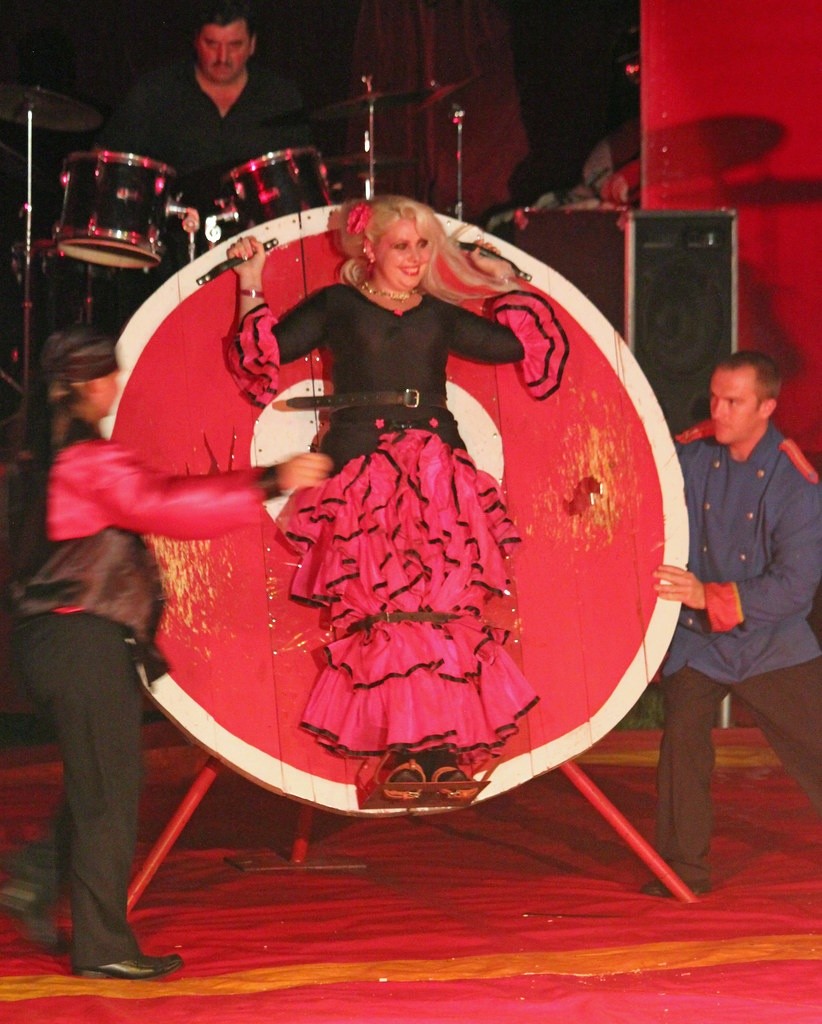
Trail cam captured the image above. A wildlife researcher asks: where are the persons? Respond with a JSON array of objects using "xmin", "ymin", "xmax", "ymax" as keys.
[
  {"xmin": 224, "ymin": 196, "xmax": 568, "ymax": 785},
  {"xmin": 90, "ymin": 0, "xmax": 312, "ymax": 202},
  {"xmin": 0, "ymin": 321, "xmax": 333, "ymax": 980},
  {"xmin": 638, "ymin": 349, "xmax": 822, "ymax": 895}
]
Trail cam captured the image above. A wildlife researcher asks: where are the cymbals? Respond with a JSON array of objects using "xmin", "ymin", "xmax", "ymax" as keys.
[
  {"xmin": 0, "ymin": 81, "xmax": 105, "ymax": 133},
  {"xmin": 409, "ymin": 66, "xmax": 490, "ymax": 118},
  {"xmin": 310, "ymin": 83, "xmax": 434, "ymax": 122},
  {"xmin": 320, "ymin": 149, "xmax": 424, "ymax": 175}
]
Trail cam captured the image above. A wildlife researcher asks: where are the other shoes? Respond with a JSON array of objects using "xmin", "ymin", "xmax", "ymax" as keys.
[
  {"xmin": 382, "ymin": 757, "xmax": 425, "ymax": 802},
  {"xmin": 428, "ymin": 766, "xmax": 477, "ymax": 798}
]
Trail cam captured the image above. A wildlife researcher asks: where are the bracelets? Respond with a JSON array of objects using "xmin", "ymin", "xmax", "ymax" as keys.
[{"xmin": 240, "ymin": 288, "xmax": 266, "ymax": 298}]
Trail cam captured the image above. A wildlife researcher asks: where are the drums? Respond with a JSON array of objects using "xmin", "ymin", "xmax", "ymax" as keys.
[
  {"xmin": 8, "ymin": 235, "xmax": 117, "ymax": 341},
  {"xmin": 54, "ymin": 148, "xmax": 178, "ymax": 270},
  {"xmin": 227, "ymin": 142, "xmax": 335, "ymax": 231},
  {"xmin": 211, "ymin": 192, "xmax": 247, "ymax": 248}
]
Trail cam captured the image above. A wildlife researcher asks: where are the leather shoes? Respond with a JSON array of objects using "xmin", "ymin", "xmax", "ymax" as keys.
[
  {"xmin": 1, "ymin": 880, "xmax": 67, "ymax": 956},
  {"xmin": 642, "ymin": 875, "xmax": 710, "ymax": 897},
  {"xmin": 78, "ymin": 951, "xmax": 181, "ymax": 980}
]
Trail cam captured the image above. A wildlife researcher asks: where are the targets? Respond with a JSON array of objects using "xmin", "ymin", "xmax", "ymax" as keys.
[{"xmin": 95, "ymin": 202, "xmax": 694, "ymax": 823}]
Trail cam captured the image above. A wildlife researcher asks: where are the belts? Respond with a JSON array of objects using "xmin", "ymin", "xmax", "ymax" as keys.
[{"xmin": 285, "ymin": 390, "xmax": 449, "ymax": 414}]
